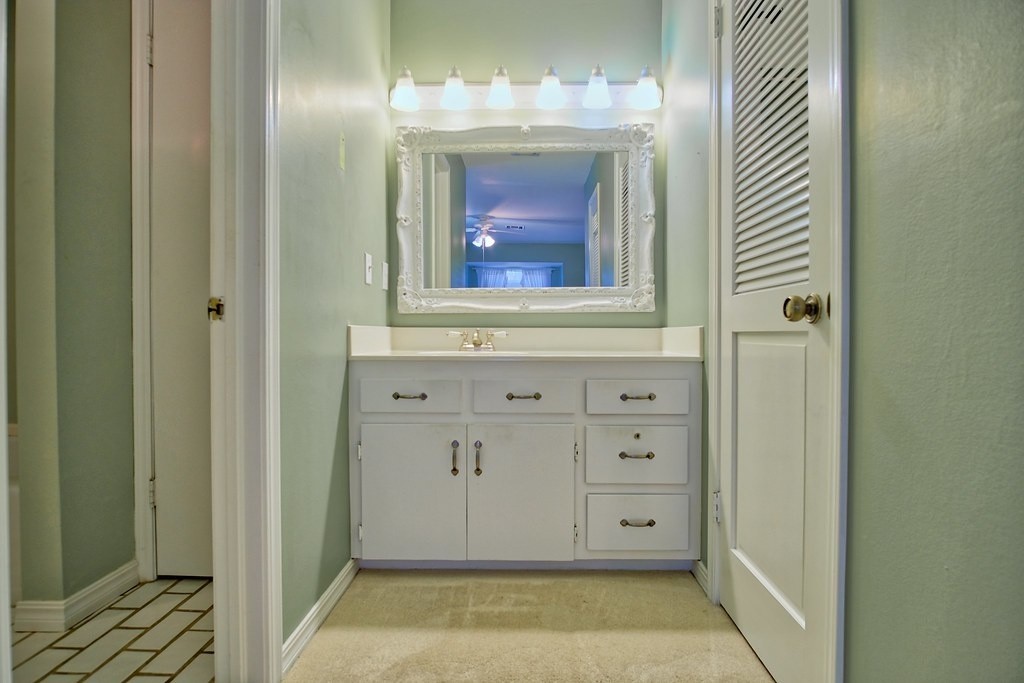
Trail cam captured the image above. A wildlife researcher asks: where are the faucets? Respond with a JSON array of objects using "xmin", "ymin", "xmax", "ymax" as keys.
[{"xmin": 472, "ymin": 330, "xmax": 482, "ymax": 346}]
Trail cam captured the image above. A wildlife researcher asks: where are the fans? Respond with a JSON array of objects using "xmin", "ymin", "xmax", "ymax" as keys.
[{"xmin": 465, "ymin": 216, "xmax": 524, "ymax": 235}]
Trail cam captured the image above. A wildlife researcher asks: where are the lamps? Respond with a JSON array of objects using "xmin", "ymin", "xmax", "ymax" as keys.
[
  {"xmin": 389, "ymin": 63, "xmax": 665, "ymax": 111},
  {"xmin": 472, "ymin": 231, "xmax": 495, "ymax": 248}
]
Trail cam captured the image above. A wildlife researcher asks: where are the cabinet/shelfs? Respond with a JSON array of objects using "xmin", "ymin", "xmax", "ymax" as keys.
[{"xmin": 346, "ymin": 358, "xmax": 702, "ymax": 573}]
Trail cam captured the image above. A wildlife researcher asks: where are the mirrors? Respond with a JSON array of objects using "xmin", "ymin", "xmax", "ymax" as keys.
[{"xmin": 396, "ymin": 122, "xmax": 656, "ymax": 314}]
